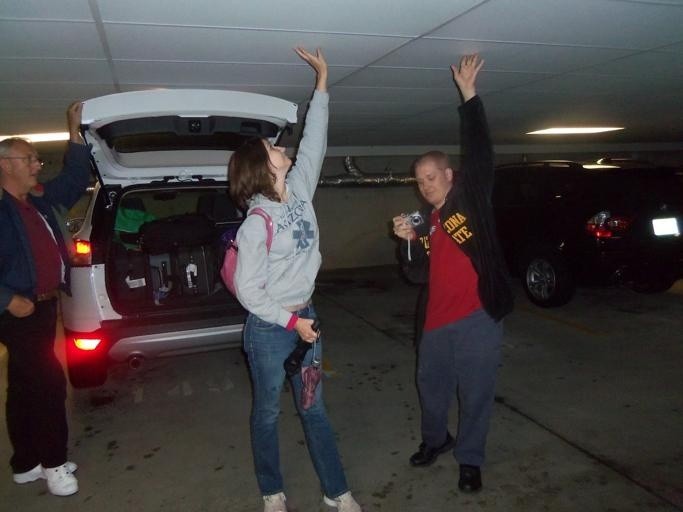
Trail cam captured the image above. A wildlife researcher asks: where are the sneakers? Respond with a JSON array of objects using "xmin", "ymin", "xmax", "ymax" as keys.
[
  {"xmin": 262, "ymin": 491, "xmax": 288, "ymax": 512},
  {"xmin": 12, "ymin": 461, "xmax": 78, "ymax": 484},
  {"xmin": 323, "ymin": 491, "xmax": 362, "ymax": 512},
  {"xmin": 41, "ymin": 463, "xmax": 78, "ymax": 497}
]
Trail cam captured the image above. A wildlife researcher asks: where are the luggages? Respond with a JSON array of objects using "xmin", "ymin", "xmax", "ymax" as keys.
[{"xmin": 106, "ymin": 206, "xmax": 217, "ymax": 310}]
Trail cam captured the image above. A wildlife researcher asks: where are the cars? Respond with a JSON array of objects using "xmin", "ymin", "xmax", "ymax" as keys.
[
  {"xmin": 51, "ymin": 88, "xmax": 302, "ymax": 390},
  {"xmin": 394, "ymin": 154, "xmax": 683, "ymax": 310}
]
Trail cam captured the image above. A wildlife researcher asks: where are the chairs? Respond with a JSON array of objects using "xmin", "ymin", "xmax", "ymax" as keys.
[
  {"xmin": 196, "ymin": 193, "xmax": 236, "ymax": 219},
  {"xmin": 119, "ymin": 198, "xmax": 146, "ymax": 212}
]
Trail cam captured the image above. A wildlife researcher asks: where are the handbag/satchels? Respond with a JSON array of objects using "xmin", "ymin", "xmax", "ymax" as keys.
[{"xmin": 220, "ymin": 207, "xmax": 273, "ymax": 298}]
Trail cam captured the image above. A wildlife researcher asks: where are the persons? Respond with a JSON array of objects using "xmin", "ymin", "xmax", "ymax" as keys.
[
  {"xmin": 0, "ymin": 101, "xmax": 92, "ymax": 497},
  {"xmin": 229, "ymin": 45, "xmax": 362, "ymax": 512},
  {"xmin": 392, "ymin": 54, "xmax": 514, "ymax": 493}
]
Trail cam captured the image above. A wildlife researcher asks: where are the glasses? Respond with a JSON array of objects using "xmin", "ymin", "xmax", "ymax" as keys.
[{"xmin": 6, "ymin": 154, "xmax": 42, "ymax": 166}]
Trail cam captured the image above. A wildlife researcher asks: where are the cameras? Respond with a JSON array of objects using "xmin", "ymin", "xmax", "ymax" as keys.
[{"xmin": 402, "ymin": 211, "xmax": 425, "ymax": 228}]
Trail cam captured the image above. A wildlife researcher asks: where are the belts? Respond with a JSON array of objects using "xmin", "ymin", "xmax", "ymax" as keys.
[{"xmin": 37, "ymin": 290, "xmax": 59, "ymax": 301}]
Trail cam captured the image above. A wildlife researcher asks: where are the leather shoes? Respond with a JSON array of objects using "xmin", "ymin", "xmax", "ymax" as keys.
[
  {"xmin": 411, "ymin": 429, "xmax": 456, "ymax": 467},
  {"xmin": 458, "ymin": 465, "xmax": 482, "ymax": 493}
]
